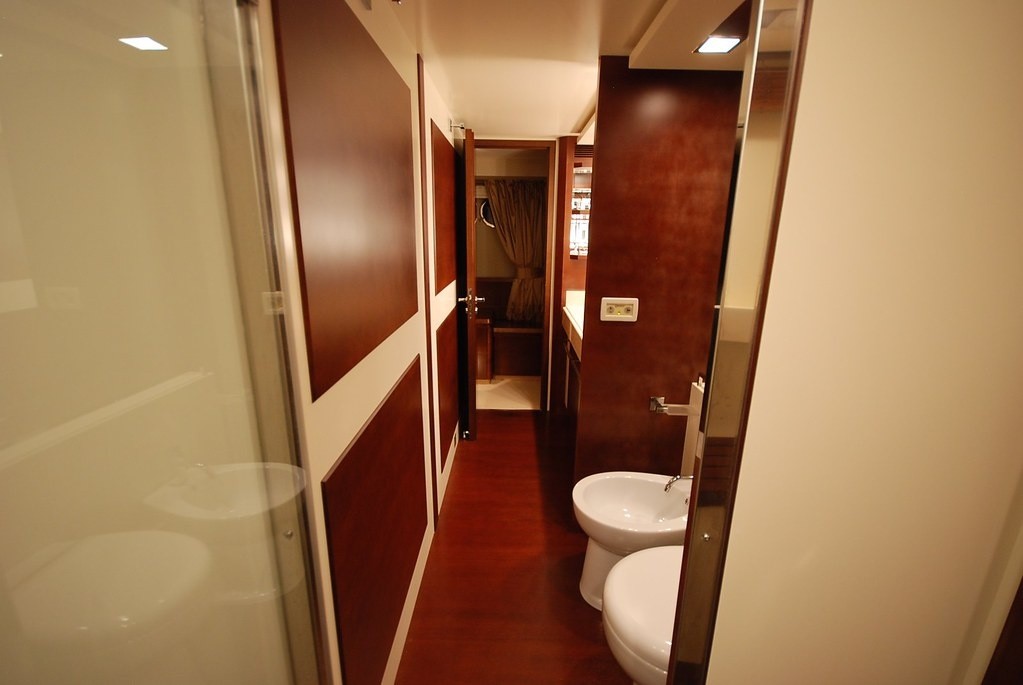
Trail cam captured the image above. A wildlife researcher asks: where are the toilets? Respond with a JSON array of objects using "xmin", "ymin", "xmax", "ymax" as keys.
[
  {"xmin": 601, "ymin": 545, "xmax": 687, "ymax": 685},
  {"xmin": 8, "ymin": 528, "xmax": 213, "ymax": 664}
]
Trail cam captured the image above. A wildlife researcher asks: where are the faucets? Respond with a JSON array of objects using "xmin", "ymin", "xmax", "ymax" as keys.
[{"xmin": 664, "ymin": 474, "xmax": 694, "ymax": 493}]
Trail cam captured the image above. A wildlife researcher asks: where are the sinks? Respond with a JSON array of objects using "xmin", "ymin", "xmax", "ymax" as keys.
[
  {"xmin": 146, "ymin": 459, "xmax": 308, "ymax": 604},
  {"xmin": 571, "ymin": 471, "xmax": 690, "ymax": 612}
]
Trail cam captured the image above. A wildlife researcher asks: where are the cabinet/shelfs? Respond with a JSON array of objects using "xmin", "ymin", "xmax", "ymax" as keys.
[{"xmin": 561, "ymin": 325, "xmax": 581, "ymax": 484}]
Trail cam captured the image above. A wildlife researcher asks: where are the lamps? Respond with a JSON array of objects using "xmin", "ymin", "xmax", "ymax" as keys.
[{"xmin": 693, "ymin": 0, "xmax": 752, "ymax": 54}]
